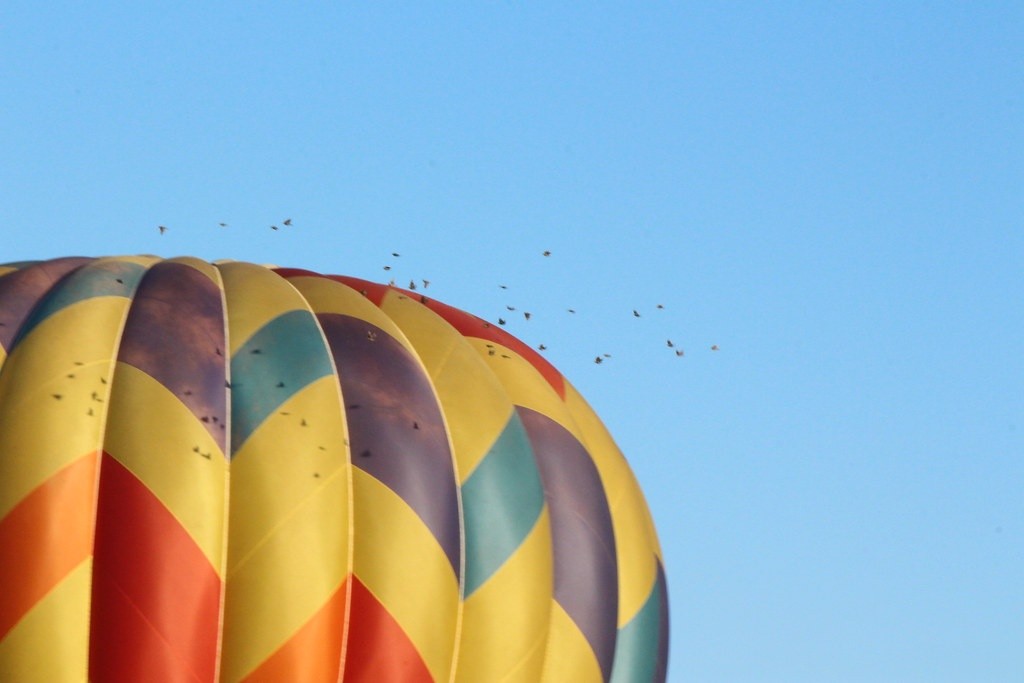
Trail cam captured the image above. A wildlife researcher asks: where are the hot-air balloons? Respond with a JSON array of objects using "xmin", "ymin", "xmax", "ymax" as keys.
[{"xmin": 1, "ymin": 253, "xmax": 671, "ymax": 683}]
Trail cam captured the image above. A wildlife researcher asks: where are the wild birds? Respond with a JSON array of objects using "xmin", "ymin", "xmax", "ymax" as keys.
[{"xmin": 0, "ymin": 216, "xmax": 721, "ymax": 481}]
